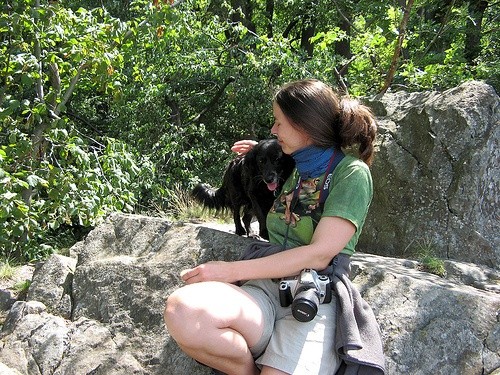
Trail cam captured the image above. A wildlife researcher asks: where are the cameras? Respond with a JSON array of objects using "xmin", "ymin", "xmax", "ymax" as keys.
[{"xmin": 279, "ymin": 268, "xmax": 332, "ymax": 322}]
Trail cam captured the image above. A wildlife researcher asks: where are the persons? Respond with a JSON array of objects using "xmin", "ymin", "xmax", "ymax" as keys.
[{"xmin": 164, "ymin": 78, "xmax": 386, "ymax": 375}]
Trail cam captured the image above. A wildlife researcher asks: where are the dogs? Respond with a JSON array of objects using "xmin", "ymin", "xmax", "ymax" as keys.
[{"xmin": 189, "ymin": 138, "xmax": 296, "ymax": 242}]
